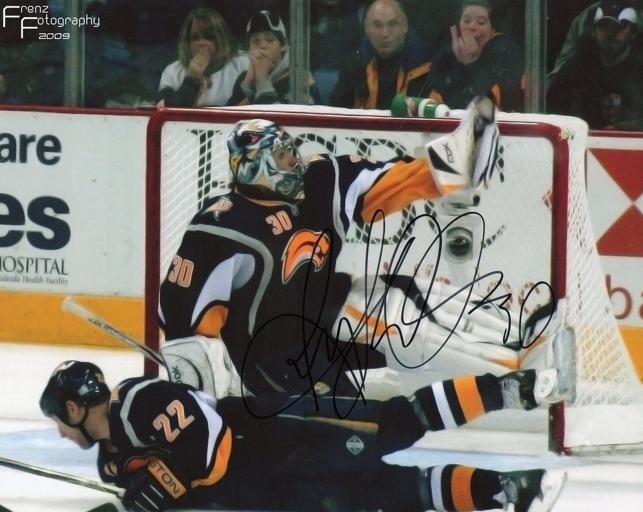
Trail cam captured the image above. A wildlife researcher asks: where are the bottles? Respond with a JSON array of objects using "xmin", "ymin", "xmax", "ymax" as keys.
[{"xmin": 391, "ymin": 94, "xmax": 451, "ymax": 118}]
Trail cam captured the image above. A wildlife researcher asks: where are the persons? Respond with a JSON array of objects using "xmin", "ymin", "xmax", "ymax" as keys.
[
  {"xmin": 428, "ymin": 0, "xmax": 519, "ymax": 111},
  {"xmin": 546, "ymin": 0, "xmax": 643, "ymax": 133},
  {"xmin": 327, "ymin": 0, "xmax": 436, "ymax": 110},
  {"xmin": 156, "ymin": 89, "xmax": 494, "ymax": 400},
  {"xmin": 38, "ymin": 359, "xmax": 575, "ymax": 512},
  {"xmin": 156, "ymin": 6, "xmax": 251, "ymax": 105},
  {"xmin": 225, "ymin": 9, "xmax": 323, "ymax": 106}
]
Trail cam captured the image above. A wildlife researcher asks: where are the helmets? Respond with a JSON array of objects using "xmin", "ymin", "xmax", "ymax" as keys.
[
  {"xmin": 39, "ymin": 360, "xmax": 111, "ymax": 417},
  {"xmin": 225, "ymin": 118, "xmax": 306, "ymax": 200}
]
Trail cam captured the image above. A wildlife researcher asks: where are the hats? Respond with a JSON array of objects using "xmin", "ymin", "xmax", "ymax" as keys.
[
  {"xmin": 241, "ymin": 9, "xmax": 289, "ymax": 46},
  {"xmin": 589, "ymin": 1, "xmax": 639, "ymax": 30}
]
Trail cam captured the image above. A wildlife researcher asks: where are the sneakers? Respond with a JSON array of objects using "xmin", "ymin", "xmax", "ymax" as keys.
[
  {"xmin": 499, "ymin": 468, "xmax": 545, "ymax": 512},
  {"xmin": 498, "ymin": 368, "xmax": 537, "ymax": 415}
]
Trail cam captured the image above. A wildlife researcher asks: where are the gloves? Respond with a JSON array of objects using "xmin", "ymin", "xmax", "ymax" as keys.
[{"xmin": 115, "ymin": 453, "xmax": 190, "ymax": 512}]
